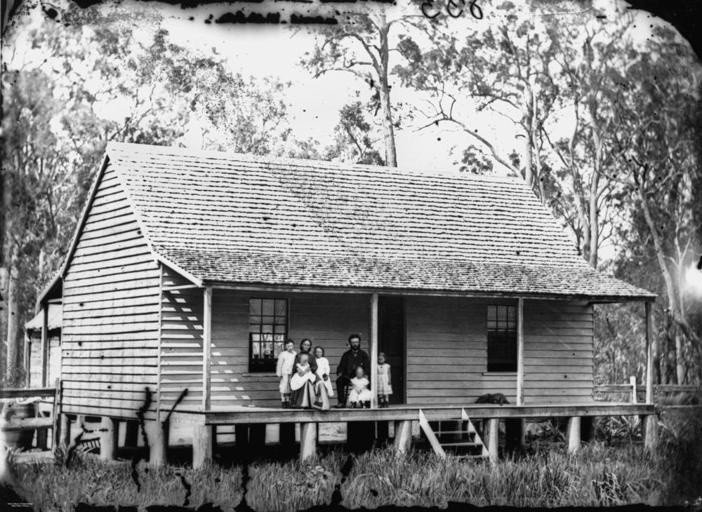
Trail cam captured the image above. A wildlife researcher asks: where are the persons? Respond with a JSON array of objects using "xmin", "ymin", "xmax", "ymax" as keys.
[
  {"xmin": 334, "ymin": 333, "xmax": 370, "ymax": 408},
  {"xmin": 295, "ymin": 353, "xmax": 310, "ymax": 377},
  {"xmin": 276, "ymin": 339, "xmax": 297, "ymax": 408},
  {"xmin": 290, "ymin": 338, "xmax": 318, "ymax": 408},
  {"xmin": 314, "ymin": 345, "xmax": 334, "ymax": 411},
  {"xmin": 378, "ymin": 351, "xmax": 394, "ymax": 407},
  {"xmin": 346, "ymin": 364, "xmax": 370, "ymax": 408}
]
